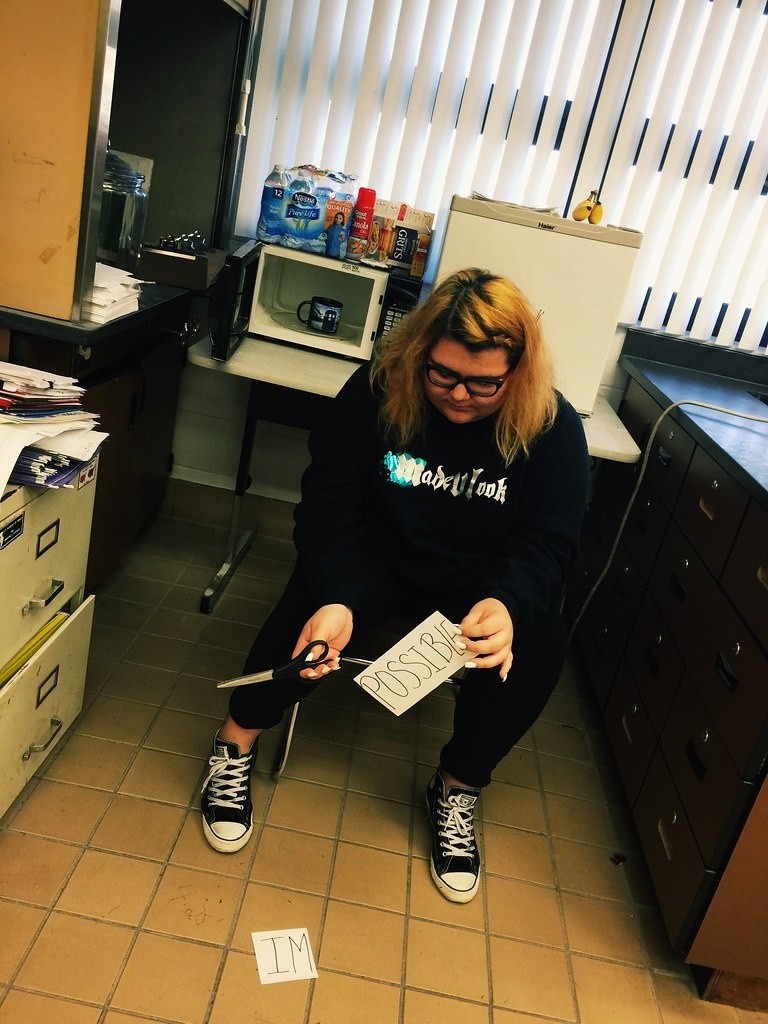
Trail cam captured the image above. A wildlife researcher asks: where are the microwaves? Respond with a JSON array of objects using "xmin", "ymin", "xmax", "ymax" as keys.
[{"xmin": 208, "ymin": 239, "xmax": 422, "ymax": 364}]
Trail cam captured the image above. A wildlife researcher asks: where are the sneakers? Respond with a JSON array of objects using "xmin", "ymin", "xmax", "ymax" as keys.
[
  {"xmin": 425, "ymin": 765, "xmax": 481, "ymax": 903},
  {"xmin": 200, "ymin": 722, "xmax": 256, "ymax": 853}
]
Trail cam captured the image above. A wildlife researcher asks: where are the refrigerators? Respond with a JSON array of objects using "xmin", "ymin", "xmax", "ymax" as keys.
[{"xmin": 431, "ymin": 193, "xmax": 643, "ymax": 416}]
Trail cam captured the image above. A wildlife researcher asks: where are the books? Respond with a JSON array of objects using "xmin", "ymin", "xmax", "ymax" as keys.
[{"xmin": 0, "ymin": 359, "xmax": 109, "ymax": 499}]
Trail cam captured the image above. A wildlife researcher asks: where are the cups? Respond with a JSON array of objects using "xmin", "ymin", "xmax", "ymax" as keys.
[{"xmin": 297, "ymin": 296, "xmax": 344, "ymax": 333}]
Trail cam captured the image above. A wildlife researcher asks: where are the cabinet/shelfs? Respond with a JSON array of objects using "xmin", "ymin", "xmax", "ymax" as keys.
[
  {"xmin": 1, "ymin": 0, "xmax": 266, "ymax": 602},
  {"xmin": 561, "ymin": 323, "xmax": 768, "ymax": 1014},
  {"xmin": 0, "ymin": 445, "xmax": 101, "ymax": 821}
]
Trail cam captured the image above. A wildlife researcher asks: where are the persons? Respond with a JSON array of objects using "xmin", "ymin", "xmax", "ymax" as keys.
[
  {"xmin": 324, "ymin": 212, "xmax": 344, "ymax": 259},
  {"xmin": 201, "ymin": 268, "xmax": 588, "ymax": 904}
]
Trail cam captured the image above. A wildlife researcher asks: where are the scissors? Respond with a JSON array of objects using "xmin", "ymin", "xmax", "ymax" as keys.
[{"xmin": 217, "ymin": 640, "xmax": 337, "ymax": 690}]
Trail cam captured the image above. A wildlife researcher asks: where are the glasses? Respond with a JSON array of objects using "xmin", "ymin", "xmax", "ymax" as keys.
[{"xmin": 423, "ymin": 353, "xmax": 519, "ymax": 397}]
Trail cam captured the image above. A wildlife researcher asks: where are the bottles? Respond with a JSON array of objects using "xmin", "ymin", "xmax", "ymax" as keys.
[
  {"xmin": 347, "ymin": 187, "xmax": 376, "ymax": 261},
  {"xmin": 96, "ymin": 139, "xmax": 149, "ymax": 275},
  {"xmin": 257, "ymin": 164, "xmax": 357, "ymax": 259}
]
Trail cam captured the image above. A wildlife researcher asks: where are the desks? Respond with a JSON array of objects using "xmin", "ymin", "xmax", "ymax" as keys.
[{"xmin": 186, "ymin": 334, "xmax": 642, "ymax": 614}]
